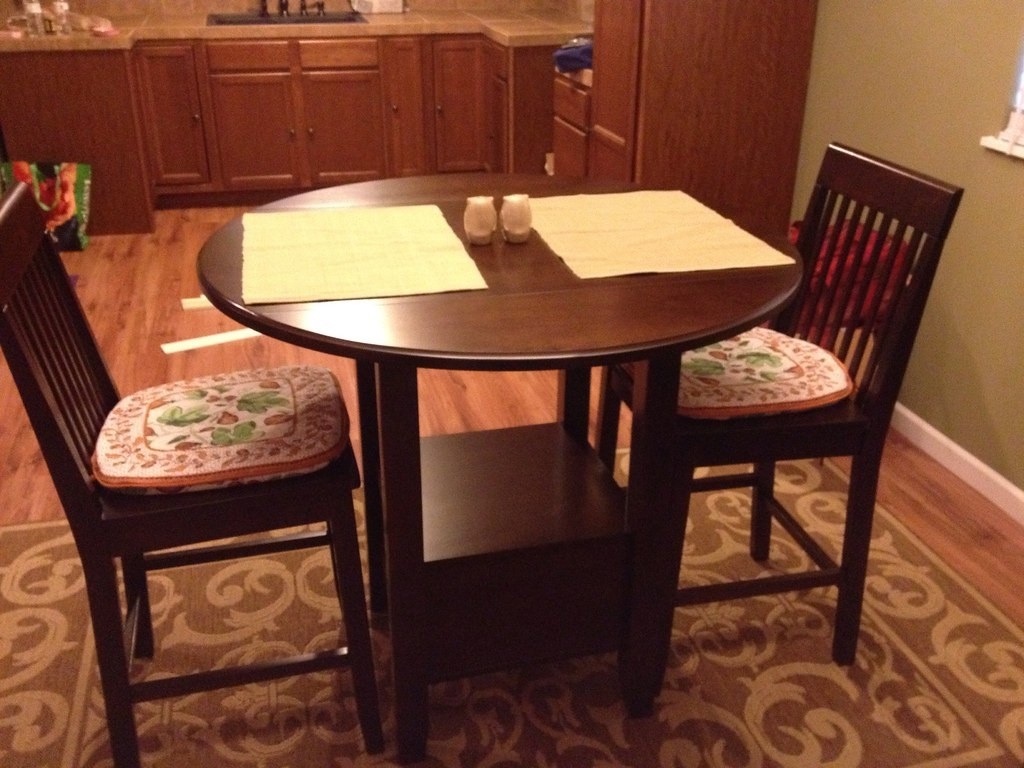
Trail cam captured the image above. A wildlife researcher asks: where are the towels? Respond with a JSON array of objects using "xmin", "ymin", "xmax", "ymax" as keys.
[{"xmin": 552, "ymin": 46, "xmax": 593, "ymax": 72}]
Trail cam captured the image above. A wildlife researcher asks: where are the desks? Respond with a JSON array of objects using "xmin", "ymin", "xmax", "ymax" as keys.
[{"xmin": 196, "ymin": 172, "xmax": 804, "ymax": 768}]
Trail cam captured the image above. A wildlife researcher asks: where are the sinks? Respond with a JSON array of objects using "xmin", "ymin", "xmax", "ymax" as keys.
[{"xmin": 205, "ymin": 11, "xmax": 370, "ymax": 24}]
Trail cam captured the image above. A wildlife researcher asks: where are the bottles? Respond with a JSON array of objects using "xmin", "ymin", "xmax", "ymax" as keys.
[
  {"xmin": 22, "ymin": 1, "xmax": 45, "ymax": 38},
  {"xmin": 500, "ymin": 194, "xmax": 531, "ymax": 243},
  {"xmin": 464, "ymin": 196, "xmax": 497, "ymax": 245},
  {"xmin": 51, "ymin": 1, "xmax": 72, "ymax": 36}
]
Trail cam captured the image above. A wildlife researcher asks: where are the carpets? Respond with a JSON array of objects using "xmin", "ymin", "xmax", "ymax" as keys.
[{"xmin": 0, "ymin": 449, "xmax": 1024, "ymax": 768}]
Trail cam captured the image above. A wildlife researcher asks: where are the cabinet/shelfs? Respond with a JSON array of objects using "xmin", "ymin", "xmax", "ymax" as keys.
[{"xmin": 0, "ymin": 0, "xmax": 820, "ymax": 245}]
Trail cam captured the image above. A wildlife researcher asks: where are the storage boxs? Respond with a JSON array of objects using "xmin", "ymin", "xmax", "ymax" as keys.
[{"xmin": 420, "ymin": 421, "xmax": 635, "ymax": 685}]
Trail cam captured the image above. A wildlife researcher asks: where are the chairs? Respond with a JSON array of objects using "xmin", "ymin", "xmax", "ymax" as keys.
[
  {"xmin": 595, "ymin": 143, "xmax": 965, "ymax": 716},
  {"xmin": 0, "ymin": 182, "xmax": 386, "ymax": 768}
]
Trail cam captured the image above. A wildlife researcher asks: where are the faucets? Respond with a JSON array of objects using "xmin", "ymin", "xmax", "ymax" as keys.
[{"xmin": 259, "ymin": 0, "xmax": 327, "ymax": 17}]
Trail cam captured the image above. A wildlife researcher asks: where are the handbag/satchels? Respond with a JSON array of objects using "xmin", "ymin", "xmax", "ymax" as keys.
[{"xmin": 0, "ymin": 160, "xmax": 91, "ymax": 250}]
